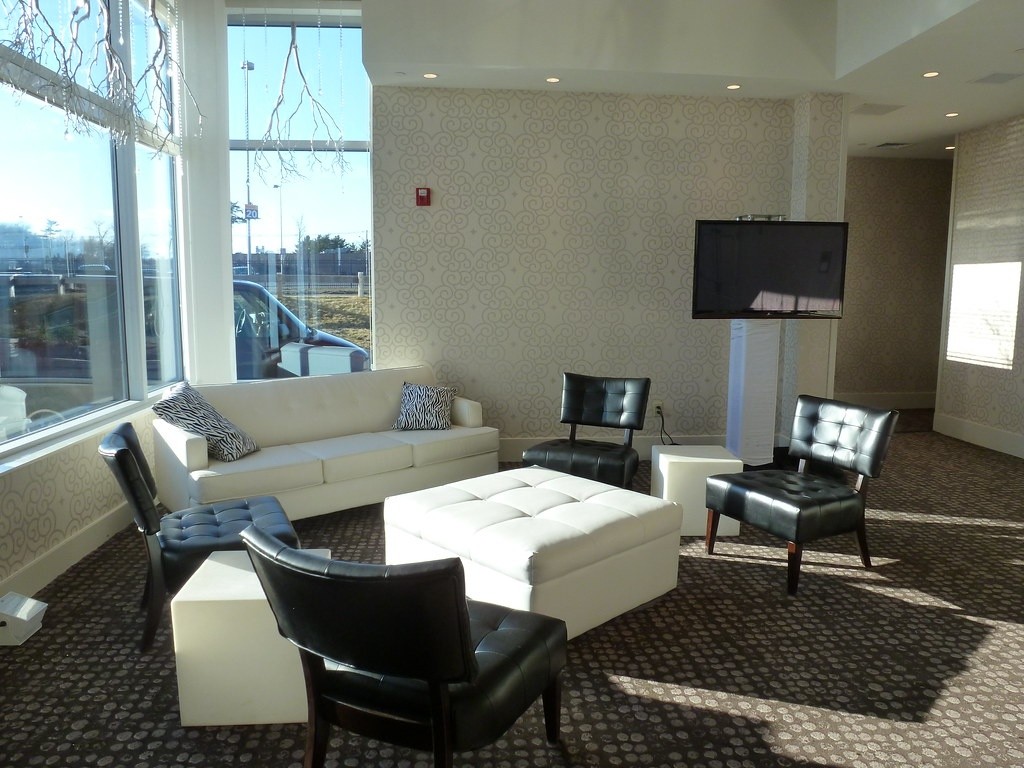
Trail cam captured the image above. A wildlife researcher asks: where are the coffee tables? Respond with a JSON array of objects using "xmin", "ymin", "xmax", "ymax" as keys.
[{"xmin": 382, "ymin": 464, "xmax": 684, "ymax": 640}]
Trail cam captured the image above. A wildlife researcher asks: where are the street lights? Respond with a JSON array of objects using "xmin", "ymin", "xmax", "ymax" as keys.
[
  {"xmin": 243, "ymin": 59, "xmax": 255, "ymax": 276},
  {"xmin": 273, "ymin": 185, "xmax": 284, "ymax": 274},
  {"xmin": 358, "ymin": 236, "xmax": 368, "ymax": 277}
]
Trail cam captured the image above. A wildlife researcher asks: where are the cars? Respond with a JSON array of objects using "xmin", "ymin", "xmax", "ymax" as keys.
[{"xmin": 15, "ymin": 264, "xmax": 256, "ymax": 276}]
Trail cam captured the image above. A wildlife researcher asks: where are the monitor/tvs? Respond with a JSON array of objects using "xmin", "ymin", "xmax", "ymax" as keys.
[{"xmin": 692, "ymin": 221, "xmax": 849, "ymax": 319}]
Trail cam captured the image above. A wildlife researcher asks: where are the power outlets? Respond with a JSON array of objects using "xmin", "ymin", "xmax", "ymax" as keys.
[{"xmin": 653, "ymin": 402, "xmax": 663, "ymax": 417}]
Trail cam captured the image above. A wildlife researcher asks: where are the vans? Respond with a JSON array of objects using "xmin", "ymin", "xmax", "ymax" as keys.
[{"xmin": 0, "ymin": 273, "xmax": 369, "ymax": 439}]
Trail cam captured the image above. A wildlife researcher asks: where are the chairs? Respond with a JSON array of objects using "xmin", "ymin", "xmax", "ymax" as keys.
[
  {"xmin": 705, "ymin": 395, "xmax": 898, "ymax": 596},
  {"xmin": 523, "ymin": 371, "xmax": 651, "ymax": 487},
  {"xmin": 237, "ymin": 522, "xmax": 568, "ymax": 768},
  {"xmin": 96, "ymin": 422, "xmax": 303, "ymax": 655}
]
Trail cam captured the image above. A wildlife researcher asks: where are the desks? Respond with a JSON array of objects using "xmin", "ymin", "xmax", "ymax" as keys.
[
  {"xmin": 169, "ymin": 546, "xmax": 331, "ymax": 726},
  {"xmin": 649, "ymin": 444, "xmax": 743, "ymax": 536}
]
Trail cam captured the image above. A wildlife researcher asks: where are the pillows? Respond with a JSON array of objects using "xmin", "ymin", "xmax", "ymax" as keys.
[
  {"xmin": 390, "ymin": 380, "xmax": 458, "ymax": 431},
  {"xmin": 150, "ymin": 381, "xmax": 261, "ymax": 463}
]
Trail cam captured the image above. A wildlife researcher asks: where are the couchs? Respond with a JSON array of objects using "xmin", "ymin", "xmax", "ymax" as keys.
[{"xmin": 148, "ymin": 364, "xmax": 500, "ymax": 521}]
